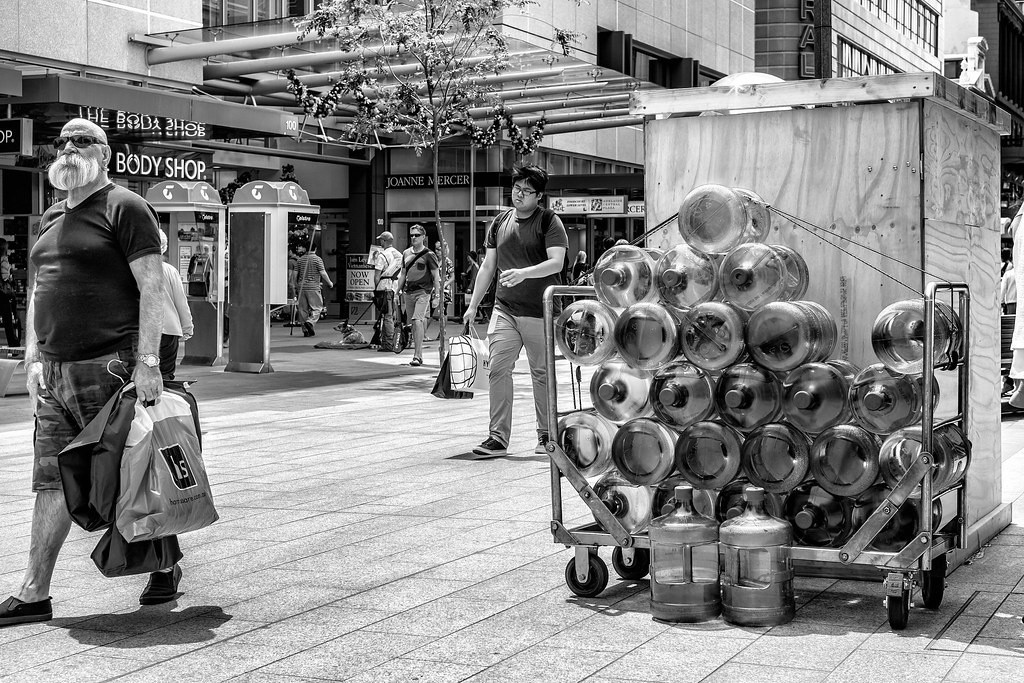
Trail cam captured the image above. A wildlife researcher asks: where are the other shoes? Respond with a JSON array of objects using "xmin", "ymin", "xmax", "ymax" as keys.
[
  {"xmin": 139, "ymin": 562, "xmax": 182, "ymax": 604},
  {"xmin": 410, "ymin": 358, "xmax": 422, "ymax": 366},
  {"xmin": 376, "ymin": 346, "xmax": 393, "ymax": 352},
  {"xmin": 304, "ymin": 322, "xmax": 315, "ymax": 338},
  {"xmin": 473, "ymin": 437, "xmax": 507, "ymax": 455},
  {"xmin": 534, "ymin": 434, "xmax": 549, "ymax": 453},
  {"xmin": 0, "ymin": 596, "xmax": 53, "ymax": 624}
]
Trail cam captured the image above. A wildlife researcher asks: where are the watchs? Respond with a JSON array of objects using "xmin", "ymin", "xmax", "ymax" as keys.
[{"xmin": 135, "ymin": 350, "xmax": 160, "ymax": 369}]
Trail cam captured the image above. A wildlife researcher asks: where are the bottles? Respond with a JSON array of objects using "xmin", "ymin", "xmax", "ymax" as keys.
[
  {"xmin": 648, "ymin": 487, "xmax": 723, "ymax": 622},
  {"xmin": 718, "ymin": 488, "xmax": 796, "ymax": 626},
  {"xmin": 553, "ymin": 189, "xmax": 972, "ymax": 551}
]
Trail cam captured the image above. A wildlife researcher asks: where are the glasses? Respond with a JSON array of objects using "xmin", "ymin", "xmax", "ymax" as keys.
[
  {"xmin": 512, "ymin": 185, "xmax": 537, "ymax": 196},
  {"xmin": 52, "ymin": 135, "xmax": 106, "ymax": 150},
  {"xmin": 410, "ymin": 233, "xmax": 422, "ymax": 237}
]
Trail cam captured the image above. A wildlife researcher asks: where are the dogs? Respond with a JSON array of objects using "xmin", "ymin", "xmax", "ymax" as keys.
[{"xmin": 333, "ymin": 319, "xmax": 368, "ymax": 344}]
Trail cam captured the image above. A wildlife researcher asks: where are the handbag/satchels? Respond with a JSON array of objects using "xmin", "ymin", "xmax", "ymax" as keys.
[
  {"xmin": 91, "ymin": 390, "xmax": 219, "ymax": 578},
  {"xmin": 392, "ymin": 302, "xmax": 408, "ymax": 353},
  {"xmin": 431, "ymin": 320, "xmax": 489, "ymax": 399}
]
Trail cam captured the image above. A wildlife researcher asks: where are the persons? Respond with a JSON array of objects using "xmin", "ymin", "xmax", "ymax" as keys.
[
  {"xmin": 1000, "ymin": 249, "xmax": 1016, "ymax": 312},
  {"xmin": 462, "ymin": 164, "xmax": 569, "ymax": 455},
  {"xmin": 559, "ymin": 236, "xmax": 631, "ymax": 306},
  {"xmin": 0, "ymin": 118, "xmax": 184, "ymax": 624},
  {"xmin": 157, "ymin": 228, "xmax": 193, "ymax": 379},
  {"xmin": 188, "ymin": 225, "xmax": 497, "ymax": 366}
]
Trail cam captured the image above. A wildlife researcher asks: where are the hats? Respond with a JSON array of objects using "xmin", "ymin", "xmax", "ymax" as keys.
[{"xmin": 376, "ymin": 231, "xmax": 394, "ymax": 241}]
